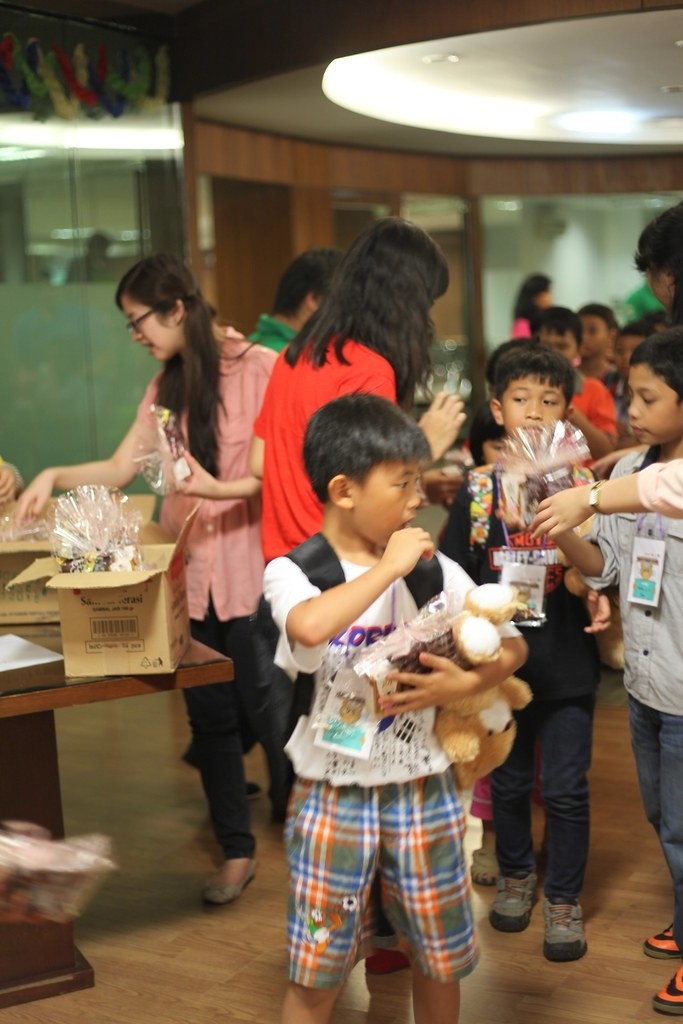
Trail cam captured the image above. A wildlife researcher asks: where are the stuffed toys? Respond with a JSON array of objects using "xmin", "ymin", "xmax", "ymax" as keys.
[{"xmin": 434, "ymin": 583, "xmax": 533, "ymax": 791}]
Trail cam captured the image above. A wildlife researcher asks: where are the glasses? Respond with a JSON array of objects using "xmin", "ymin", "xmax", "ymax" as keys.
[{"xmin": 125, "ymin": 307, "xmax": 160, "ymax": 334}]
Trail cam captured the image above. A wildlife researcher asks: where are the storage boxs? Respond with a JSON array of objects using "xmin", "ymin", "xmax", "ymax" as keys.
[
  {"xmin": 0, "ymin": 493, "xmax": 157, "ymax": 624},
  {"xmin": 3, "ymin": 497, "xmax": 208, "ymax": 678}
]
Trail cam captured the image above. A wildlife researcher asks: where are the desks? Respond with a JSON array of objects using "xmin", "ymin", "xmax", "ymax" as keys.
[{"xmin": 0, "ymin": 619, "xmax": 240, "ymax": 1011}]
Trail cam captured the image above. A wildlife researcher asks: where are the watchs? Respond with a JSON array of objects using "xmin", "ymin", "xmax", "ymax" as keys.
[{"xmin": 589, "ymin": 479, "xmax": 612, "ymax": 514}]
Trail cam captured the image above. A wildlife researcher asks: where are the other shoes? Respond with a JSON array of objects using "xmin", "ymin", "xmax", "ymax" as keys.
[
  {"xmin": 240, "ymin": 780, "xmax": 259, "ymax": 802},
  {"xmin": 200, "ymin": 856, "xmax": 260, "ymax": 905},
  {"xmin": 364, "ymin": 946, "xmax": 409, "ymax": 974},
  {"xmin": 471, "ymin": 846, "xmax": 500, "ymax": 885}
]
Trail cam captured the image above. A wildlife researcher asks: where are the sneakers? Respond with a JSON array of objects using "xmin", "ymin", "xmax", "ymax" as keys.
[
  {"xmin": 643, "ymin": 922, "xmax": 683, "ymax": 959},
  {"xmin": 652, "ymin": 963, "xmax": 683, "ymax": 1016},
  {"xmin": 488, "ymin": 871, "xmax": 538, "ymax": 933},
  {"xmin": 542, "ymin": 899, "xmax": 588, "ymax": 963}
]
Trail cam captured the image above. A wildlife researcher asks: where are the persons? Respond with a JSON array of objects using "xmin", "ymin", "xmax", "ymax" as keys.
[
  {"xmin": 510, "ymin": 202, "xmax": 683, "ymax": 477},
  {"xmin": 14, "ymin": 253, "xmax": 281, "ymax": 906},
  {"xmin": 546, "ymin": 330, "xmax": 683, "ymax": 1016},
  {"xmin": 247, "ymin": 217, "xmax": 467, "ymax": 824},
  {"xmin": 262, "ymin": 394, "xmax": 528, "ymax": 1024},
  {"xmin": 528, "ymin": 458, "xmax": 683, "ymax": 543},
  {"xmin": 436, "ymin": 337, "xmax": 612, "ymax": 963}
]
